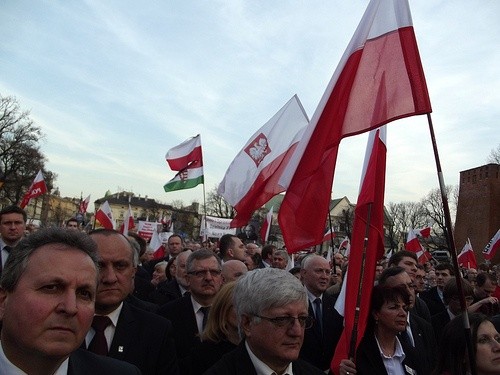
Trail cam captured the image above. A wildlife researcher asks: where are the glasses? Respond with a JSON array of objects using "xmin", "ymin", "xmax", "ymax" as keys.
[{"xmin": 244, "ymin": 311, "xmax": 315, "ymax": 329}]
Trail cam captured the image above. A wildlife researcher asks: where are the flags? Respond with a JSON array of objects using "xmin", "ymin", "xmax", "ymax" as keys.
[
  {"xmin": 324, "ymin": 212, "xmax": 336, "ymax": 241},
  {"xmin": 480, "ymin": 229, "xmax": 500, "ymax": 259},
  {"xmin": 94, "ymin": 200, "xmax": 117, "ymax": 230},
  {"xmin": 20, "ymin": 170, "xmax": 49, "ymax": 210},
  {"xmin": 405, "ymin": 225, "xmax": 422, "ymax": 254},
  {"xmin": 123, "ymin": 204, "xmax": 134, "ymax": 237},
  {"xmin": 164, "ymin": 134, "xmax": 204, "ymax": 193},
  {"xmin": 218, "ymin": 94, "xmax": 309, "ymax": 227},
  {"xmin": 278, "ymin": 0, "xmax": 441, "ymax": 262},
  {"xmin": 329, "ymin": 124, "xmax": 387, "ymax": 375},
  {"xmin": 413, "ymin": 225, "xmax": 431, "ymax": 236},
  {"xmin": 147, "ymin": 228, "xmax": 166, "ymax": 260},
  {"xmin": 257, "ymin": 205, "xmax": 274, "ymax": 245},
  {"xmin": 456, "ymin": 237, "xmax": 477, "ymax": 268},
  {"xmin": 416, "ymin": 242, "xmax": 433, "ymax": 264}
]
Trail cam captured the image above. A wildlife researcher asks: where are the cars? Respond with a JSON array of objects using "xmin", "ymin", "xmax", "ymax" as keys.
[{"xmin": 431, "ymin": 250, "xmax": 450, "ymax": 261}]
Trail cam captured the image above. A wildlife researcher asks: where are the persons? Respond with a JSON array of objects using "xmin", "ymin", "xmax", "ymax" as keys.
[{"xmin": 0, "ymin": 205, "xmax": 500, "ymax": 375}]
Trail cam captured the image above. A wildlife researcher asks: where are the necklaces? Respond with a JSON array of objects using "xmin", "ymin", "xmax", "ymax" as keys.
[{"xmin": 388, "ymin": 353, "xmax": 392, "ymax": 357}]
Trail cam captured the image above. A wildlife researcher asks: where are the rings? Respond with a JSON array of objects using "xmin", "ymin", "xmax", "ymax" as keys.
[{"xmin": 347, "ymin": 372, "xmax": 349, "ymax": 375}]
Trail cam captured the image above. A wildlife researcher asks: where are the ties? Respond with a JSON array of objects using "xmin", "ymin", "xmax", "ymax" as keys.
[
  {"xmin": 312, "ymin": 297, "xmax": 323, "ymax": 335},
  {"xmin": 198, "ymin": 306, "xmax": 210, "ymax": 332},
  {"xmin": 87, "ymin": 315, "xmax": 113, "ymax": 356},
  {"xmin": 3, "ymin": 246, "xmax": 14, "ymax": 254},
  {"xmin": 183, "ymin": 291, "xmax": 191, "ymax": 305}
]
